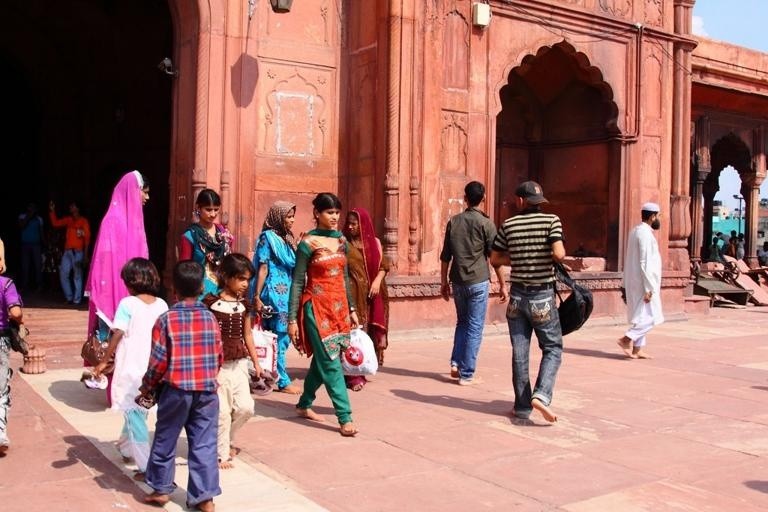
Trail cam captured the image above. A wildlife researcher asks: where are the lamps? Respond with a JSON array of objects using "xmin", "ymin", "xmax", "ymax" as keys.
[{"xmin": 248, "ymin": 0, "xmax": 293, "ymax": 19}]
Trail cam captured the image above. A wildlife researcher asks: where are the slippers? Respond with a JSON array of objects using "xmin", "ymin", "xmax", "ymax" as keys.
[{"xmin": 249, "ymin": 375, "xmax": 274, "ymax": 396}]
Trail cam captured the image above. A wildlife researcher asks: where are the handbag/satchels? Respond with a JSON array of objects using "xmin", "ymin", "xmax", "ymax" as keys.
[
  {"xmin": 338, "ymin": 327, "xmax": 381, "ymax": 377},
  {"xmin": 554, "ymin": 258, "xmax": 594, "ymax": 336},
  {"xmin": 6, "ymin": 316, "xmax": 30, "ymax": 356},
  {"xmin": 80, "ymin": 331, "xmax": 116, "ymax": 375},
  {"xmin": 243, "ymin": 313, "xmax": 280, "ymax": 383}
]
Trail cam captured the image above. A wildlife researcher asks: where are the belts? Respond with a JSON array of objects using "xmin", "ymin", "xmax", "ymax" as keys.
[
  {"xmin": 511, "ymin": 281, "xmax": 555, "ymax": 292},
  {"xmin": 65, "ymin": 248, "xmax": 76, "ymax": 257}
]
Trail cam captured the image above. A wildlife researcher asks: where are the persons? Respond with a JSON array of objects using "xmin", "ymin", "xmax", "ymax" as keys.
[
  {"xmin": 440, "ymin": 181, "xmax": 507, "ymax": 385},
  {"xmin": 491, "ymin": 181, "xmax": 591, "ymax": 423},
  {"xmin": 709, "ymin": 230, "xmax": 768, "ymax": 267},
  {"xmin": 617, "ymin": 203, "xmax": 666, "ymax": 358}
]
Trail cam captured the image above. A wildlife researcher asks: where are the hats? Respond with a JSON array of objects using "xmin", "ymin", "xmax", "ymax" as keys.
[
  {"xmin": 513, "ymin": 180, "xmax": 550, "ymax": 206},
  {"xmin": 640, "ymin": 202, "xmax": 660, "ymax": 212}
]
